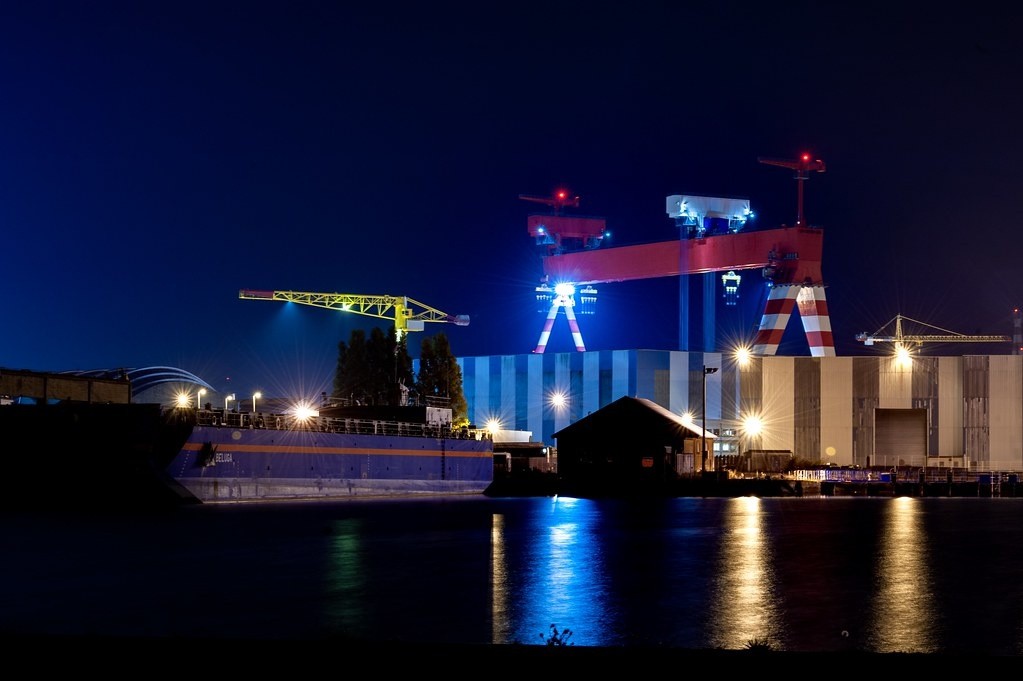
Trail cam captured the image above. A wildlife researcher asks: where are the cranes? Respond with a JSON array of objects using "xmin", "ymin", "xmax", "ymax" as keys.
[
  {"xmin": 854, "ymin": 312, "xmax": 1015, "ymax": 357},
  {"xmin": 236, "ymin": 287, "xmax": 471, "ymax": 349}
]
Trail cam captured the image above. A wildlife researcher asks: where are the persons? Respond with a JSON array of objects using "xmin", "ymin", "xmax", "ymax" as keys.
[{"xmin": 193, "ymin": 407, "xmax": 466, "ymax": 439}]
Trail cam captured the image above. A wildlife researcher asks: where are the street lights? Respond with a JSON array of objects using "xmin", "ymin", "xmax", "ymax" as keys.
[
  {"xmin": 198, "ymin": 388, "xmax": 207, "ymax": 409},
  {"xmin": 224, "ymin": 395, "xmax": 232, "ymax": 409},
  {"xmin": 702, "ymin": 363, "xmax": 719, "ymax": 499},
  {"xmin": 252, "ymin": 392, "xmax": 262, "ymax": 412}
]
